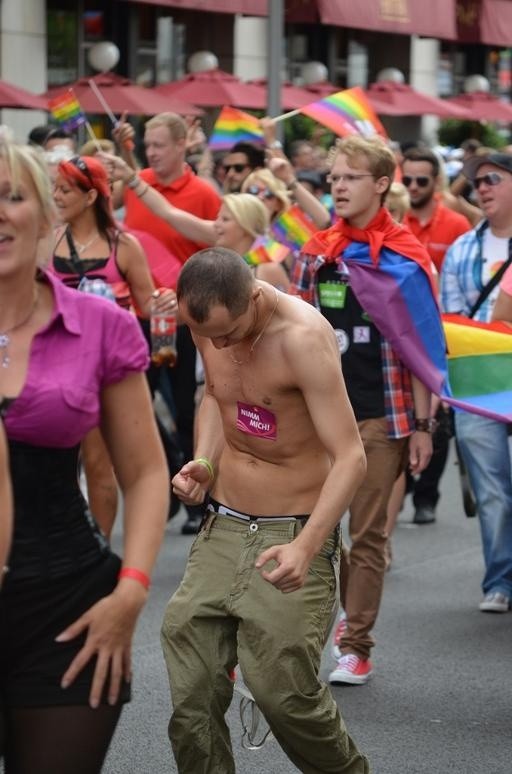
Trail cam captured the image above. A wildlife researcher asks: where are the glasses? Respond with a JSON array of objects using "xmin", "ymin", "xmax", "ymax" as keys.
[
  {"xmin": 69, "ymin": 157, "xmax": 92, "ymax": 185},
  {"xmin": 247, "ymin": 184, "xmax": 276, "ymax": 200},
  {"xmin": 474, "ymin": 172, "xmax": 505, "ymax": 188},
  {"xmin": 223, "ymin": 163, "xmax": 249, "ymax": 173},
  {"xmin": 326, "ymin": 174, "xmax": 376, "ymax": 183},
  {"xmin": 401, "ymin": 176, "xmax": 428, "ymax": 187}
]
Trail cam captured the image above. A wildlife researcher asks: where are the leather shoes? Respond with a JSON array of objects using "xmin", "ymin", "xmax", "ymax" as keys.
[{"xmin": 181, "ymin": 513, "xmax": 204, "ymax": 533}]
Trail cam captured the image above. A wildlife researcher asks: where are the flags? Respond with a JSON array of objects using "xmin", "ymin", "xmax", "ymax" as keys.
[
  {"xmin": 208, "ymin": 104, "xmax": 267, "ymax": 150},
  {"xmin": 45, "ymin": 85, "xmax": 87, "ymax": 132},
  {"xmin": 303, "ymin": 84, "xmax": 395, "ymax": 149}
]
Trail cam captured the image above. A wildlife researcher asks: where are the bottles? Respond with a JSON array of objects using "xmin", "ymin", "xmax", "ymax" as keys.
[{"xmin": 149, "ymin": 287, "xmax": 179, "ymax": 368}]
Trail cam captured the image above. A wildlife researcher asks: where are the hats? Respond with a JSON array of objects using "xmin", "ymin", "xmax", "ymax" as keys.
[{"xmin": 463, "ymin": 154, "xmax": 511, "ymax": 180}]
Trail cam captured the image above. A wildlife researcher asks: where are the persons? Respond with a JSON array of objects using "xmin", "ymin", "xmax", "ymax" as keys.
[
  {"xmin": 158, "ymin": 246, "xmax": 377, "ymax": 773},
  {"xmin": 1, "ymin": 111, "xmax": 509, "ymax": 686},
  {"xmin": 0, "ymin": 137, "xmax": 169, "ymax": 774}
]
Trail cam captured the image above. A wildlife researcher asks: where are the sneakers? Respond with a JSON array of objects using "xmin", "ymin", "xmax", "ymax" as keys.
[
  {"xmin": 414, "ymin": 507, "xmax": 435, "ymax": 523},
  {"xmin": 328, "ymin": 612, "xmax": 373, "ymax": 684},
  {"xmin": 479, "ymin": 591, "xmax": 509, "ymax": 612}
]
